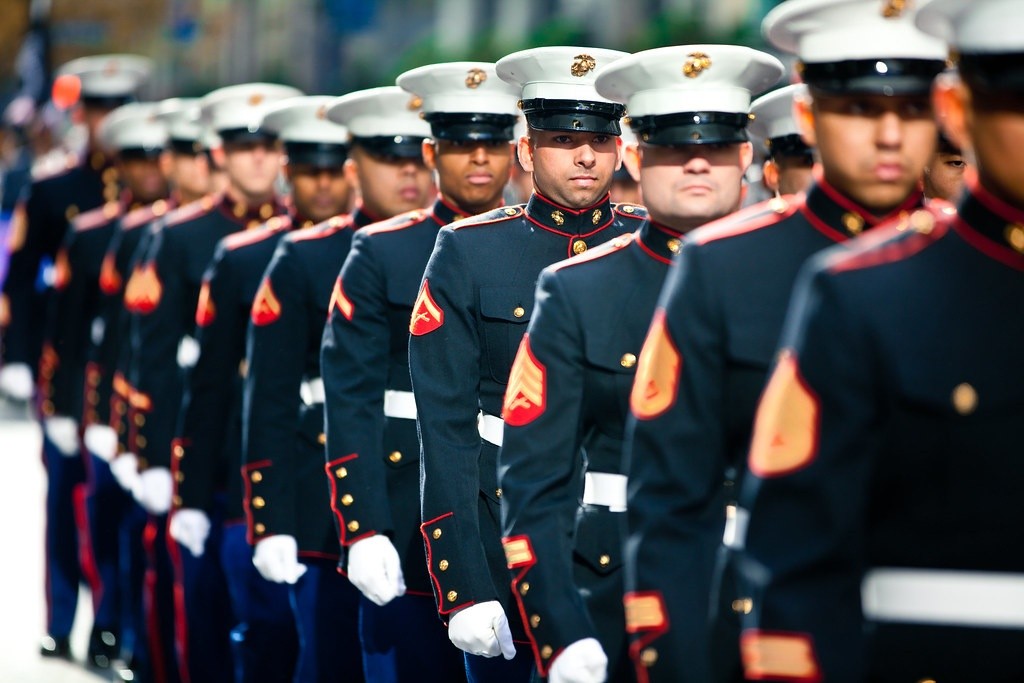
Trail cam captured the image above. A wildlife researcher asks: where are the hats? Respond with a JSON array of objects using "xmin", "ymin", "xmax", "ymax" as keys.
[
  {"xmin": 94, "ymin": 102, "xmax": 165, "ymax": 156},
  {"xmin": 150, "ymin": 97, "xmax": 208, "ymax": 154},
  {"xmin": 594, "ymin": 44, "xmax": 786, "ymax": 145},
  {"xmin": 916, "ymin": 0, "xmax": 1023, "ymax": 110},
  {"xmin": 324, "ymin": 86, "xmax": 435, "ymax": 157},
  {"xmin": 396, "ymin": 62, "xmax": 519, "ymax": 140},
  {"xmin": 262, "ymin": 95, "xmax": 351, "ymax": 168},
  {"xmin": 199, "ymin": 82, "xmax": 305, "ymax": 146},
  {"xmin": 58, "ymin": 51, "xmax": 153, "ymax": 106},
  {"xmin": 497, "ymin": 46, "xmax": 630, "ymax": 136},
  {"xmin": 750, "ymin": 83, "xmax": 809, "ymax": 156},
  {"xmin": 761, "ymin": 0, "xmax": 951, "ymax": 96}
]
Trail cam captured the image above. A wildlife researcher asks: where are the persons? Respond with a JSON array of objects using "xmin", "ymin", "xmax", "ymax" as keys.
[
  {"xmin": 497, "ymin": 38, "xmax": 790, "ymax": 681},
  {"xmin": 165, "ymin": 99, "xmax": 356, "ymax": 683},
  {"xmin": 749, "ymin": 79, "xmax": 820, "ymax": 201},
  {"xmin": 319, "ymin": 61, "xmax": 524, "ymax": 683},
  {"xmin": 241, "ymin": 79, "xmax": 437, "ymax": 679},
  {"xmin": 617, "ymin": 0, "xmax": 961, "ymax": 681},
  {"xmin": 914, "ymin": 131, "xmax": 977, "ymax": 204},
  {"xmin": 0, "ymin": 0, "xmax": 305, "ymax": 683},
  {"xmin": 406, "ymin": 43, "xmax": 647, "ymax": 682},
  {"xmin": 741, "ymin": 0, "xmax": 1023, "ymax": 683}
]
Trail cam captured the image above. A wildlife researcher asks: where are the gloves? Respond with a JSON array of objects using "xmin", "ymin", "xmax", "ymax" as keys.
[
  {"xmin": 82, "ymin": 422, "xmax": 117, "ymax": 460},
  {"xmin": 133, "ymin": 468, "xmax": 174, "ymax": 516},
  {"xmin": 109, "ymin": 453, "xmax": 140, "ymax": 492},
  {"xmin": 547, "ymin": 639, "xmax": 606, "ymax": 683},
  {"xmin": 45, "ymin": 417, "xmax": 77, "ymax": 455},
  {"xmin": 3, "ymin": 361, "xmax": 32, "ymax": 399},
  {"xmin": 348, "ymin": 535, "xmax": 407, "ymax": 606},
  {"xmin": 170, "ymin": 508, "xmax": 212, "ymax": 557},
  {"xmin": 253, "ymin": 536, "xmax": 307, "ymax": 585},
  {"xmin": 447, "ymin": 600, "xmax": 517, "ymax": 661}
]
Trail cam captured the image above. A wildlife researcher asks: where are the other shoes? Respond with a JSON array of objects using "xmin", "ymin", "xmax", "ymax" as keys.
[
  {"xmin": 41, "ymin": 638, "xmax": 70, "ymax": 656},
  {"xmin": 91, "ymin": 647, "xmax": 117, "ymax": 669}
]
